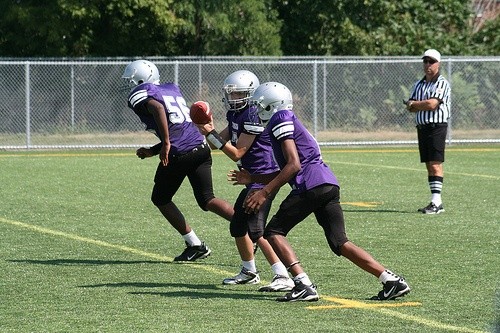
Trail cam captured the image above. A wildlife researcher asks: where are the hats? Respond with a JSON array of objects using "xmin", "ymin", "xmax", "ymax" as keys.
[{"xmin": 421, "ymin": 49, "xmax": 440, "ymax": 62}]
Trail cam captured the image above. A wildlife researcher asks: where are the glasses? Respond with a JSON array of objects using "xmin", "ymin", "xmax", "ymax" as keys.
[{"xmin": 422, "ymin": 60, "xmax": 435, "ymax": 64}]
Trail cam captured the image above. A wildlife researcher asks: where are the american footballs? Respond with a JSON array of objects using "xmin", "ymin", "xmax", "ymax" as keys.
[{"xmin": 189, "ymin": 100, "xmax": 213, "ymax": 125}]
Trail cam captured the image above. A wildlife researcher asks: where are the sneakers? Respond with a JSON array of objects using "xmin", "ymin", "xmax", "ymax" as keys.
[
  {"xmin": 174, "ymin": 240, "xmax": 211, "ymax": 261},
  {"xmin": 257, "ymin": 275, "xmax": 295, "ymax": 292},
  {"xmin": 371, "ymin": 277, "xmax": 411, "ymax": 300},
  {"xmin": 253, "ymin": 242, "xmax": 258, "ymax": 254},
  {"xmin": 223, "ymin": 267, "xmax": 260, "ymax": 285},
  {"xmin": 276, "ymin": 283, "xmax": 319, "ymax": 301}
]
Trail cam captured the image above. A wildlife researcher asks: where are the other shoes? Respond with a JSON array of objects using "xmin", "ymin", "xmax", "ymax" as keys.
[{"xmin": 418, "ymin": 202, "xmax": 445, "ymax": 214}]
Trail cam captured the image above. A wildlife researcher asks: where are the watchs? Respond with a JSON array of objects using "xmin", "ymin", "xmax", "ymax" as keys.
[{"xmin": 409, "ymin": 101, "xmax": 414, "ymax": 110}]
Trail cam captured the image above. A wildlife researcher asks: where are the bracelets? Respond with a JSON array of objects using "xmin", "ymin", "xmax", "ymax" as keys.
[
  {"xmin": 262, "ymin": 189, "xmax": 274, "ymax": 201},
  {"xmin": 206, "ymin": 128, "xmax": 227, "ymax": 150}
]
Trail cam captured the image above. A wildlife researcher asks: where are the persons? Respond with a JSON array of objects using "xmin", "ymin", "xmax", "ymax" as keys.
[
  {"xmin": 195, "ymin": 70, "xmax": 295, "ymax": 292},
  {"xmin": 403, "ymin": 48, "xmax": 451, "ymax": 214},
  {"xmin": 243, "ymin": 81, "xmax": 411, "ymax": 302},
  {"xmin": 122, "ymin": 60, "xmax": 258, "ymax": 262}
]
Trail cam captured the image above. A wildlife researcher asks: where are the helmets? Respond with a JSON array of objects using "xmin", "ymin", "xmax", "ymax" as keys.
[
  {"xmin": 248, "ymin": 82, "xmax": 293, "ymax": 128},
  {"xmin": 221, "ymin": 70, "xmax": 259, "ymax": 111},
  {"xmin": 121, "ymin": 60, "xmax": 160, "ymax": 85}
]
